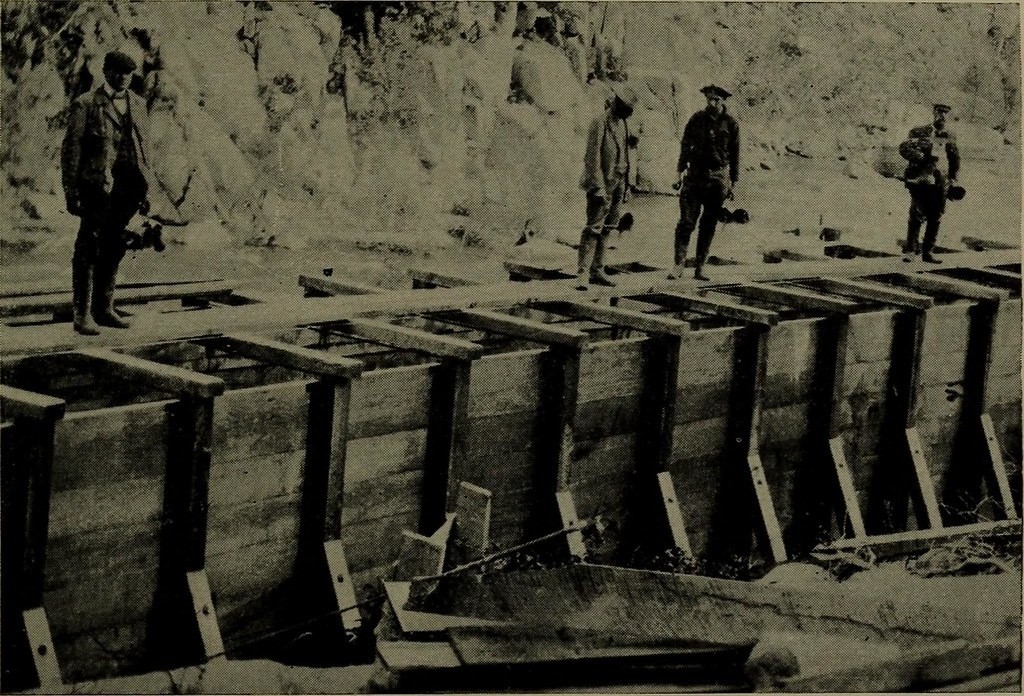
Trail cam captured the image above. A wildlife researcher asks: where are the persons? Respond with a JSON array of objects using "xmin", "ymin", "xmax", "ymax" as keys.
[
  {"xmin": 60, "ymin": 51, "xmax": 147, "ymax": 336},
  {"xmin": 667, "ymin": 86, "xmax": 742, "ymax": 282},
  {"xmin": 899, "ymin": 104, "xmax": 961, "ymax": 265},
  {"xmin": 574, "ymin": 87, "xmax": 636, "ymax": 291}
]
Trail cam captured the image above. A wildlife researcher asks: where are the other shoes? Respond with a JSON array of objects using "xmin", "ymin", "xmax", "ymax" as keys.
[
  {"xmin": 695, "ymin": 262, "xmax": 710, "ymax": 281},
  {"xmin": 922, "ymin": 253, "xmax": 943, "ymax": 264},
  {"xmin": 575, "ymin": 273, "xmax": 588, "ymax": 291},
  {"xmin": 589, "ymin": 276, "xmax": 616, "ymax": 287},
  {"xmin": 903, "ymin": 251, "xmax": 914, "ymax": 262},
  {"xmin": 667, "ymin": 265, "xmax": 682, "ymax": 279}
]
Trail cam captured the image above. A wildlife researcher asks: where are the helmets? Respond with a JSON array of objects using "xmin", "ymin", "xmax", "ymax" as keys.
[{"xmin": 732, "ymin": 209, "xmax": 749, "ymax": 223}]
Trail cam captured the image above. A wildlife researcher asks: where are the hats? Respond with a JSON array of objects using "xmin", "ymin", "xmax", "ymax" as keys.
[
  {"xmin": 610, "ymin": 84, "xmax": 637, "ymax": 108},
  {"xmin": 103, "ymin": 51, "xmax": 137, "ymax": 71},
  {"xmin": 699, "ymin": 84, "xmax": 732, "ymax": 100},
  {"xmin": 933, "ymin": 103, "xmax": 952, "ymax": 113}
]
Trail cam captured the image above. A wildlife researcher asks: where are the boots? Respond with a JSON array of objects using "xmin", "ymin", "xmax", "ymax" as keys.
[
  {"xmin": 91, "ymin": 273, "xmax": 130, "ymax": 328},
  {"xmin": 72, "ymin": 270, "xmax": 100, "ymax": 335}
]
